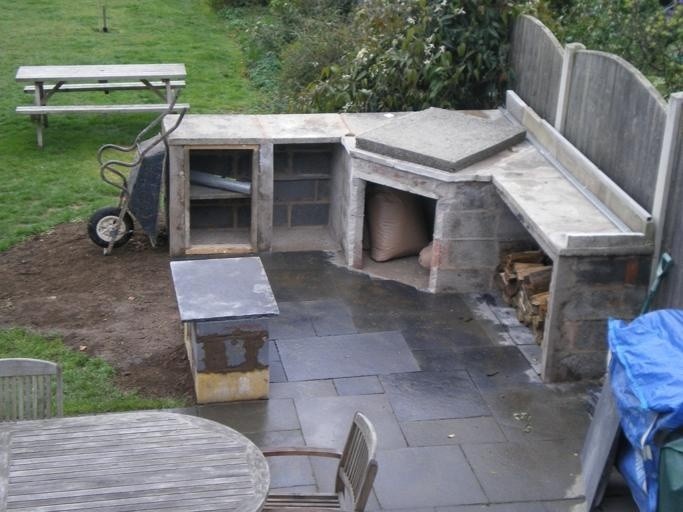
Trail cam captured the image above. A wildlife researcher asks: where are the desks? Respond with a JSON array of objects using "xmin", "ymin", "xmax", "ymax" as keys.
[{"xmin": 14, "ymin": 61, "xmax": 188, "ymax": 148}]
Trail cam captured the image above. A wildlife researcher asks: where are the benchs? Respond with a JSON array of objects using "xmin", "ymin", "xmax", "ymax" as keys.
[{"xmin": 13, "ymin": 77, "xmax": 191, "ymax": 148}]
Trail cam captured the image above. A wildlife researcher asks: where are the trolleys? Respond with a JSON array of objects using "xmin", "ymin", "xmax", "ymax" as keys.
[{"xmin": 85, "ymin": 85, "xmax": 190, "ymax": 258}]
